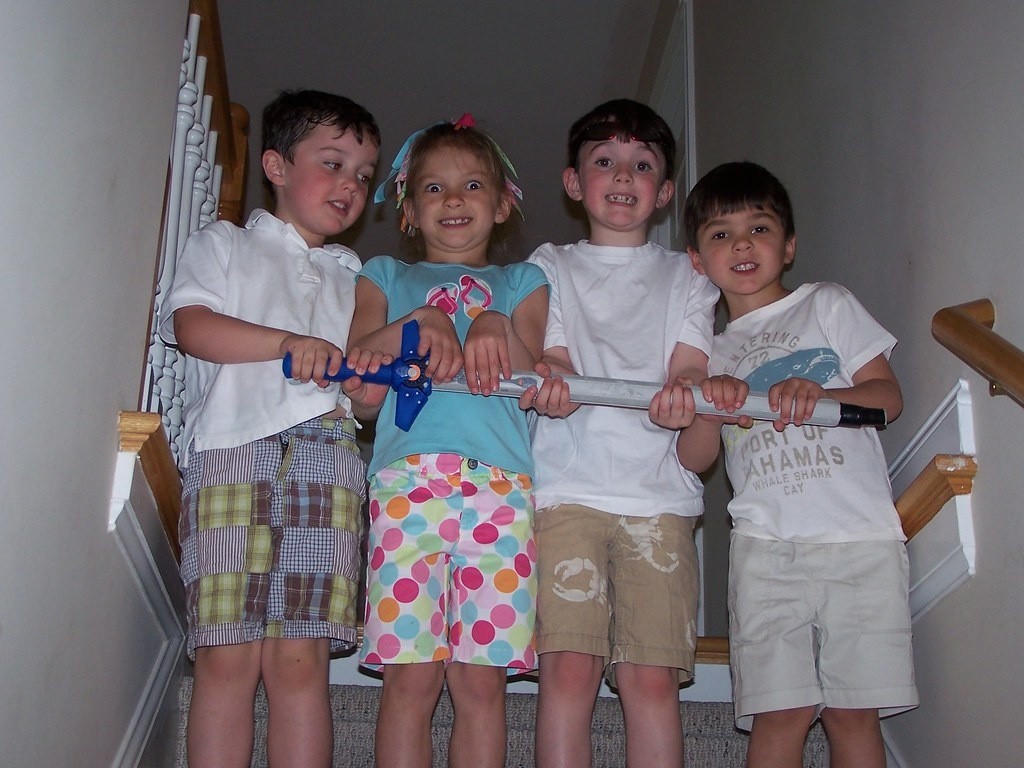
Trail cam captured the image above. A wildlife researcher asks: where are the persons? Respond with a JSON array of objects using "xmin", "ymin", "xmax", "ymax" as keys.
[
  {"xmin": 676, "ymin": 162, "xmax": 921, "ymax": 768},
  {"xmin": 343, "ymin": 115, "xmax": 552, "ymax": 768},
  {"xmin": 518, "ymin": 98, "xmax": 723, "ymax": 768},
  {"xmin": 157, "ymin": 86, "xmax": 393, "ymax": 767}
]
